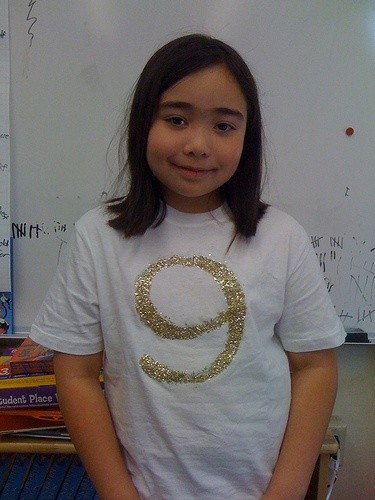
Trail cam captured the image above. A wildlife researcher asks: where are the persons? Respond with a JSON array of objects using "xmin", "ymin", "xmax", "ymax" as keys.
[{"xmin": 27, "ymin": 32, "xmax": 348, "ymax": 500}]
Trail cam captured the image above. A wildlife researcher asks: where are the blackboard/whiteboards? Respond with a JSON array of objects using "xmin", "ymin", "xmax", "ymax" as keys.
[{"xmin": 1, "ymin": 1, "xmax": 373, "ymax": 345}]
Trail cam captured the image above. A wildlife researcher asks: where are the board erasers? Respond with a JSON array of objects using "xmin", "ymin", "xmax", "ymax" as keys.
[{"xmin": 343, "ymin": 327, "xmax": 369, "ymax": 343}]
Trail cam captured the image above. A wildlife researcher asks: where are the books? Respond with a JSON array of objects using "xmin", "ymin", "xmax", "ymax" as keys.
[{"xmin": 0, "ymin": 334, "xmax": 106, "ymax": 456}]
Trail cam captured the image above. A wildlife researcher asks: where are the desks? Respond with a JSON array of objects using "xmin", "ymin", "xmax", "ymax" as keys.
[{"xmin": 1, "ymin": 405, "xmax": 339, "ymax": 500}]
